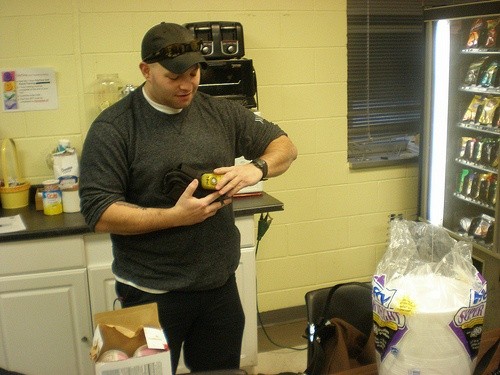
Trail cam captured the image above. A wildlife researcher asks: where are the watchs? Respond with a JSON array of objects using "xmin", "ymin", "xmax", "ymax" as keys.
[{"xmin": 250, "ymin": 159, "xmax": 268, "ymax": 180}]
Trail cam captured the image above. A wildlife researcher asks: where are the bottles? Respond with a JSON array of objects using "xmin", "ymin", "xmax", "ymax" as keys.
[
  {"xmin": 42, "ymin": 179, "xmax": 63, "ymax": 215},
  {"xmin": 122, "ymin": 83, "xmax": 136, "ymax": 98},
  {"xmin": 95, "ymin": 72, "xmax": 124, "ymax": 114}
]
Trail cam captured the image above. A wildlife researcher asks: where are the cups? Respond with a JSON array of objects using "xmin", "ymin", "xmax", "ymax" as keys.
[{"xmin": 62, "ymin": 190, "xmax": 81, "ymax": 213}]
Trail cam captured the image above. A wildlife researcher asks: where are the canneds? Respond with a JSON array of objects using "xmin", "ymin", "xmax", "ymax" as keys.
[
  {"xmin": 41, "ymin": 179, "xmax": 64, "ymax": 215},
  {"xmin": 97, "ymin": 344, "xmax": 163, "ymax": 363}
]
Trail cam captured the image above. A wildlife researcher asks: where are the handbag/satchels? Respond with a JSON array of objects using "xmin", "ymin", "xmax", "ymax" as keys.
[{"xmin": 303, "ymin": 282, "xmax": 381, "ymax": 375}]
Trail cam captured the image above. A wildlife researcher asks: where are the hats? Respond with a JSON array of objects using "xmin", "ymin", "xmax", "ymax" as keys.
[{"xmin": 141, "ymin": 22, "xmax": 209, "ymax": 74}]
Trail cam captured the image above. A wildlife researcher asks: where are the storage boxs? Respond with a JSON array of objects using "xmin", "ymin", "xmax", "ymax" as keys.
[
  {"xmin": 91, "ymin": 302, "xmax": 171, "ymax": 375},
  {"xmin": 53, "ymin": 151, "xmax": 78, "ymax": 178}
]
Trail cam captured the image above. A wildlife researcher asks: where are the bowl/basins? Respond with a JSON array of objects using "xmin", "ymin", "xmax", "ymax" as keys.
[{"xmin": 0, "ymin": 180, "xmax": 31, "ymax": 209}]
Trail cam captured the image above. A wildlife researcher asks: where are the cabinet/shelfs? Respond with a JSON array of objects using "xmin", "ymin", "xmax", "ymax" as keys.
[
  {"xmin": 84, "ymin": 214, "xmax": 258, "ymax": 367},
  {"xmin": 0, "ymin": 234, "xmax": 95, "ymax": 375}
]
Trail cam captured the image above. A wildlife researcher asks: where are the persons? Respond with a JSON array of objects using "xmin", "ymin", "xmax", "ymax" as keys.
[{"xmin": 78, "ymin": 22, "xmax": 297, "ymax": 375}]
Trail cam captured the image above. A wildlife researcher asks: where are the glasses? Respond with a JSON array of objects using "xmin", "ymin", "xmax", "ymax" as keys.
[{"xmin": 142, "ymin": 39, "xmax": 204, "ymax": 61}]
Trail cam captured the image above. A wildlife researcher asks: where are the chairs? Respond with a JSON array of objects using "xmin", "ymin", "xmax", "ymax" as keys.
[{"xmin": 304, "ymin": 281, "xmax": 372, "ymax": 375}]
